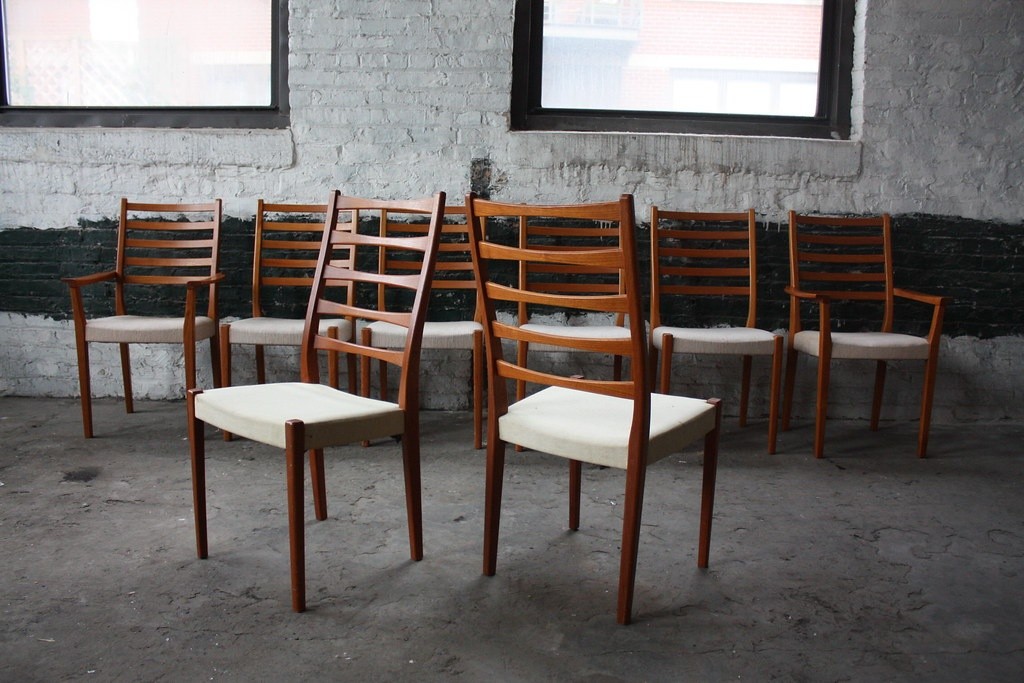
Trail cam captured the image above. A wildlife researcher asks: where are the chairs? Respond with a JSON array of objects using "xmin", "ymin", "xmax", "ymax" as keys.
[
  {"xmin": 187, "ymin": 190, "xmax": 446, "ymax": 613},
  {"xmin": 222, "ymin": 198, "xmax": 359, "ymax": 440},
  {"xmin": 361, "ymin": 206, "xmax": 487, "ymax": 450},
  {"xmin": 61, "ymin": 198, "xmax": 220, "ymax": 440},
  {"xmin": 515, "ymin": 200, "xmax": 625, "ymax": 470},
  {"xmin": 649, "ymin": 206, "xmax": 781, "ymax": 454},
  {"xmin": 465, "ymin": 193, "xmax": 722, "ymax": 625},
  {"xmin": 782, "ymin": 210, "xmax": 944, "ymax": 458}
]
